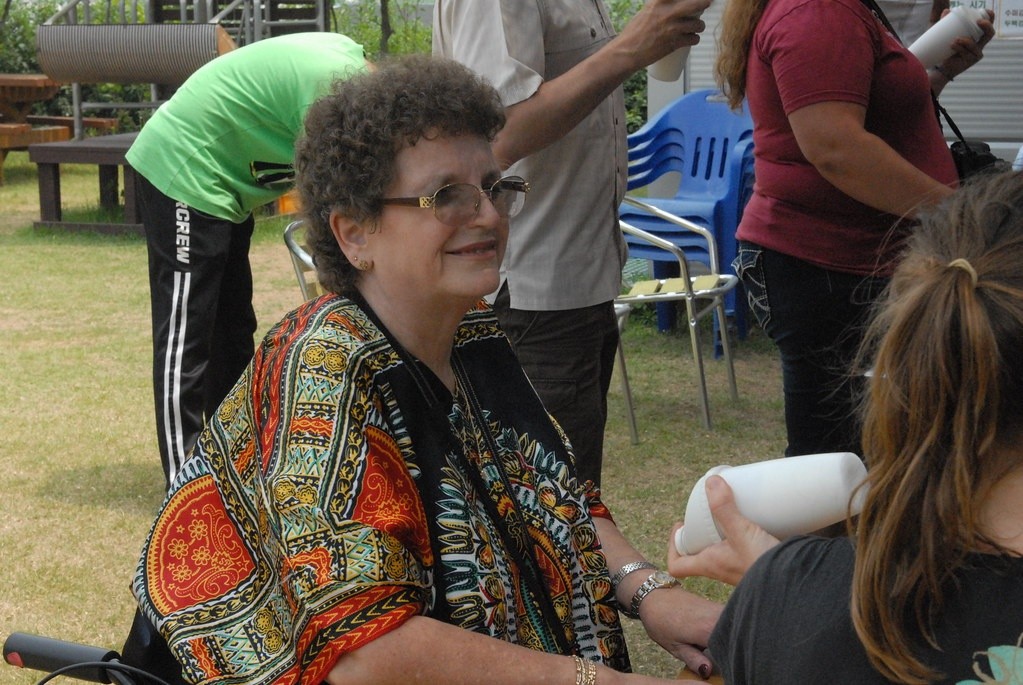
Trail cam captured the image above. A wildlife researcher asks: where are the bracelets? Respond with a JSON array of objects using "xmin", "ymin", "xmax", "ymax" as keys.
[
  {"xmin": 571, "ymin": 654, "xmax": 596, "ymax": 684},
  {"xmin": 611, "ymin": 561, "xmax": 659, "ymax": 594},
  {"xmin": 935, "ymin": 64, "xmax": 954, "ymax": 82}
]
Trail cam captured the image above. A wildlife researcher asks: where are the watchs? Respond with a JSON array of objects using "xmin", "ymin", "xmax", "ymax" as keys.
[{"xmin": 630, "ymin": 571, "xmax": 682, "ymax": 618}]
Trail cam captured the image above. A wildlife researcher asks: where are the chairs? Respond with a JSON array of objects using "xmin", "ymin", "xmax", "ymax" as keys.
[
  {"xmin": 279, "ymin": 221, "xmax": 334, "ymax": 301},
  {"xmin": 613, "ymin": 86, "xmax": 765, "ymax": 443}
]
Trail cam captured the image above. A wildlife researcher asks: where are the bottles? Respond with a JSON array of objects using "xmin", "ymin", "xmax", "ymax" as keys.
[
  {"xmin": 674, "ymin": 453, "xmax": 871, "ymax": 559},
  {"xmin": 906, "ymin": 6, "xmax": 992, "ymax": 80},
  {"xmin": 647, "ymin": 11, "xmax": 699, "ymax": 82}
]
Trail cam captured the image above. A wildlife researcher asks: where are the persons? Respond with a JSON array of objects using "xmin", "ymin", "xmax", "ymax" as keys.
[
  {"xmin": 117, "ymin": 57, "xmax": 726, "ymax": 684},
  {"xmin": 432, "ymin": 0, "xmax": 710, "ymax": 498},
  {"xmin": 123, "ymin": 32, "xmax": 380, "ymax": 495},
  {"xmin": 670, "ymin": 171, "xmax": 1023, "ymax": 685},
  {"xmin": 715, "ymin": 0, "xmax": 996, "ymax": 463}
]
